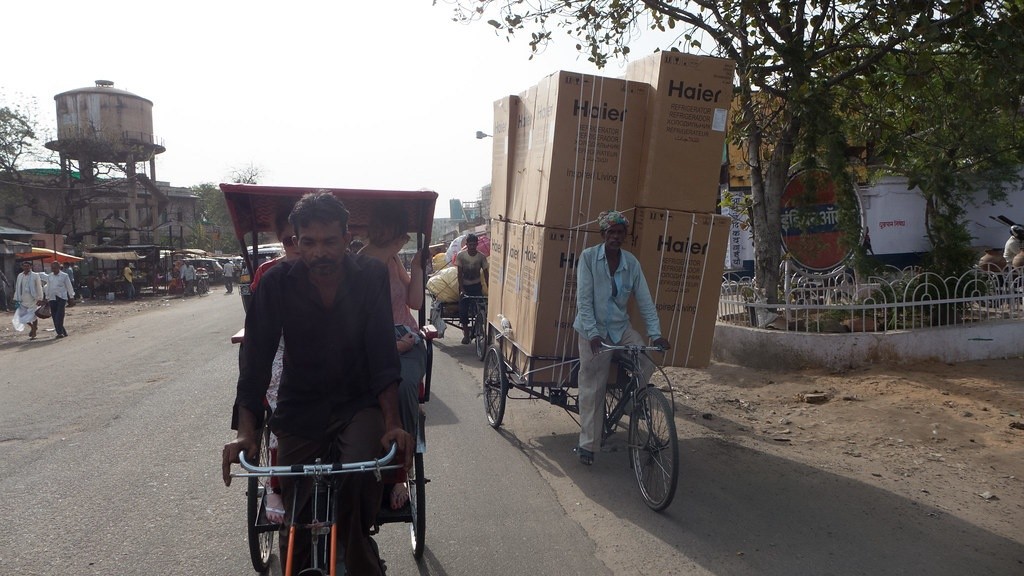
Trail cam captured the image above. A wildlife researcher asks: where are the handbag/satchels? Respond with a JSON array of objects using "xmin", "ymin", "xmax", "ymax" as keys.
[{"xmin": 35, "ymin": 303, "xmax": 52, "ymax": 319}]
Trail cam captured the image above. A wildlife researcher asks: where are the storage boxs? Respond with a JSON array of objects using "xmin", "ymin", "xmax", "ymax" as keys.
[{"xmin": 486, "ymin": 50, "xmax": 735, "ymax": 370}]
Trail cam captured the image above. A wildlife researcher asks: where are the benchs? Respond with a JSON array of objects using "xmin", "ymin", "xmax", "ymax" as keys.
[{"xmin": 233, "ymin": 325, "xmax": 430, "ymax": 411}]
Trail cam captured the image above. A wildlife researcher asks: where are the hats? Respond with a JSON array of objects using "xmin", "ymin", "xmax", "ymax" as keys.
[{"xmin": 597, "ymin": 209, "xmax": 630, "ymax": 231}]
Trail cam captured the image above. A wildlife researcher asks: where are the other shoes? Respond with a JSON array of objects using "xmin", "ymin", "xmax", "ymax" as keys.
[
  {"xmin": 461, "ymin": 336, "xmax": 469, "ymax": 344},
  {"xmin": 29, "ymin": 327, "xmax": 38, "ymax": 340},
  {"xmin": 56, "ymin": 332, "xmax": 68, "ymax": 338}
]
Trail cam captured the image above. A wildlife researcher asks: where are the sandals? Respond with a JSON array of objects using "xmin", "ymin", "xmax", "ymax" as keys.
[
  {"xmin": 390, "ymin": 485, "xmax": 409, "ymax": 509},
  {"xmin": 264, "ymin": 495, "xmax": 285, "ymax": 525},
  {"xmin": 577, "ymin": 446, "xmax": 594, "ymax": 466},
  {"xmin": 624, "ymin": 405, "xmax": 649, "ymax": 419}
]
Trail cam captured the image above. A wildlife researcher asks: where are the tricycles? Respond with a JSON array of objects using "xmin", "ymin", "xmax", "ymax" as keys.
[
  {"xmin": 218, "ymin": 180, "xmax": 439, "ymax": 576},
  {"xmin": 426, "ymin": 290, "xmax": 490, "ymax": 363},
  {"xmin": 480, "ymin": 321, "xmax": 682, "ymax": 511}
]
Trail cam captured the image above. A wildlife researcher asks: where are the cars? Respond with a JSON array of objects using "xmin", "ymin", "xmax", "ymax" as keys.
[{"xmin": 160, "ymin": 242, "xmax": 289, "ymax": 314}]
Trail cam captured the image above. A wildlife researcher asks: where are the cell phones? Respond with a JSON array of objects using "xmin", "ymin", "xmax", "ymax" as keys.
[{"xmin": 395, "ymin": 324, "xmax": 409, "ymax": 338}]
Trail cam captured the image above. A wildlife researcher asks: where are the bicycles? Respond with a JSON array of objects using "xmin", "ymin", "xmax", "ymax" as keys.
[{"xmin": 195, "ymin": 272, "xmax": 209, "ymax": 297}]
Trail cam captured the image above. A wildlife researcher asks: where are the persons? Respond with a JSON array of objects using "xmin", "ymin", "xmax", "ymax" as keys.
[
  {"xmin": 14, "ymin": 262, "xmax": 44, "ymax": 339},
  {"xmin": 63, "ymin": 262, "xmax": 75, "ymax": 306},
  {"xmin": 359, "ymin": 209, "xmax": 431, "ymax": 508},
  {"xmin": 122, "ymin": 262, "xmax": 137, "ymax": 301},
  {"xmin": 979, "ymin": 225, "xmax": 1024, "ymax": 286},
  {"xmin": 433, "ymin": 248, "xmax": 441, "ymax": 257},
  {"xmin": 221, "ymin": 193, "xmax": 416, "ymax": 576},
  {"xmin": 455, "ymin": 234, "xmax": 488, "ymax": 344},
  {"xmin": 172, "ymin": 260, "xmax": 196, "ymax": 297},
  {"xmin": 863, "ymin": 227, "xmax": 874, "ymax": 255},
  {"xmin": 571, "ymin": 210, "xmax": 671, "ymax": 466},
  {"xmin": 46, "ymin": 262, "xmax": 75, "ymax": 338},
  {"xmin": 94, "ymin": 271, "xmax": 109, "ymax": 289},
  {"xmin": 250, "ymin": 200, "xmax": 301, "ymax": 525},
  {"xmin": 222, "ymin": 259, "xmax": 233, "ymax": 292}
]
